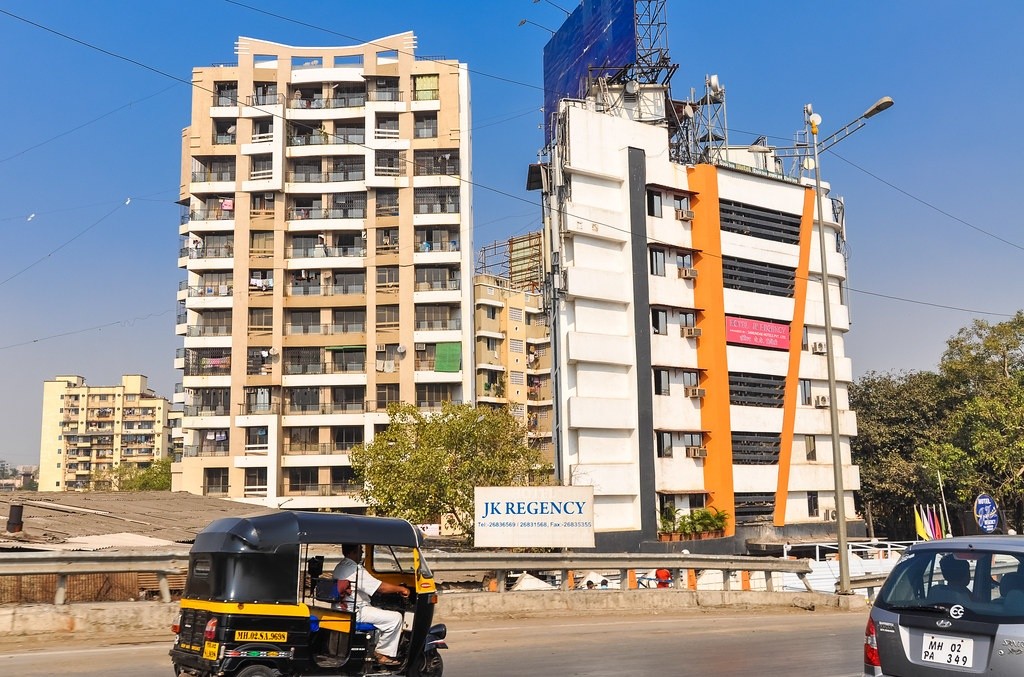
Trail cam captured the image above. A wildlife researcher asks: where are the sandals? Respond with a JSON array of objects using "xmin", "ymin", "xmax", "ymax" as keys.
[{"xmin": 373, "ymin": 652, "xmax": 402, "ymax": 664}]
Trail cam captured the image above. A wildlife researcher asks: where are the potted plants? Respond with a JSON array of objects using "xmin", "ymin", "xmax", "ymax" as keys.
[{"xmin": 655, "ymin": 502, "xmax": 728, "ymax": 541}]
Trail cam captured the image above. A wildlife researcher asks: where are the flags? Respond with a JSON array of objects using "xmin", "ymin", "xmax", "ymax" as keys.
[{"xmin": 914, "ymin": 503, "xmax": 946, "ymax": 542}]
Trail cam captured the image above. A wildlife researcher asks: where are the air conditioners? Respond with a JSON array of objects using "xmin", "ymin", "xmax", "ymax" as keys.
[
  {"xmin": 687, "ymin": 447, "xmax": 707, "ymax": 458},
  {"xmin": 689, "ymin": 387, "xmax": 706, "ymax": 398},
  {"xmin": 265, "ymin": 193, "xmax": 273, "ymax": 199},
  {"xmin": 377, "ymin": 79, "xmax": 385, "ymax": 85},
  {"xmin": 817, "ymin": 396, "xmax": 831, "ymax": 406},
  {"xmin": 685, "ymin": 327, "xmax": 701, "ymax": 337},
  {"xmin": 680, "ymin": 210, "xmax": 694, "ymax": 220},
  {"xmin": 813, "ymin": 342, "xmax": 827, "ymax": 353},
  {"xmin": 377, "ymin": 344, "xmax": 385, "ymax": 351},
  {"xmin": 681, "ymin": 269, "xmax": 697, "ymax": 278},
  {"xmin": 415, "ymin": 343, "xmax": 425, "ymax": 350},
  {"xmin": 824, "ymin": 508, "xmax": 837, "ymax": 521}
]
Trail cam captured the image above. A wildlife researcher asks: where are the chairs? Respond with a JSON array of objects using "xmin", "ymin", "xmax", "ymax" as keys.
[{"xmin": 316, "ymin": 579, "xmax": 379, "ymax": 630}]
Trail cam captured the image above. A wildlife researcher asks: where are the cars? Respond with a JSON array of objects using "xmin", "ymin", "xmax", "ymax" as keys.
[{"xmin": 864, "ymin": 535, "xmax": 1024, "ymax": 677}]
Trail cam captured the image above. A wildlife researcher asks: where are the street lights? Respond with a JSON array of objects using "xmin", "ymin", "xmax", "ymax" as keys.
[{"xmin": 747, "ymin": 95, "xmax": 895, "ymax": 597}]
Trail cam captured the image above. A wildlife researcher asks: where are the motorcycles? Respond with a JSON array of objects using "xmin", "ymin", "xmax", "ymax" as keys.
[{"xmin": 168, "ymin": 511, "xmax": 450, "ymax": 677}]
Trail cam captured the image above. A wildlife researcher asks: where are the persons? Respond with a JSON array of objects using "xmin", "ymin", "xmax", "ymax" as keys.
[
  {"xmin": 332, "ymin": 543, "xmax": 410, "ymax": 665},
  {"xmin": 939, "ymin": 554, "xmax": 972, "ymax": 598},
  {"xmin": 582, "ymin": 580, "xmax": 596, "ymax": 590},
  {"xmin": 599, "ymin": 579, "xmax": 609, "ymax": 590},
  {"xmin": 655, "ymin": 569, "xmax": 672, "ymax": 588}
]
